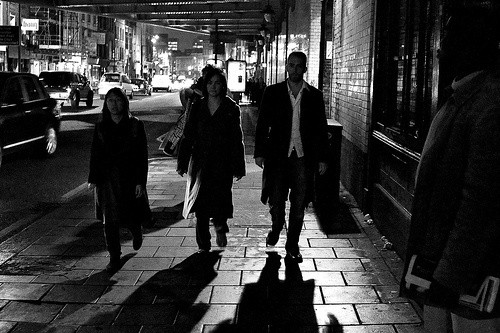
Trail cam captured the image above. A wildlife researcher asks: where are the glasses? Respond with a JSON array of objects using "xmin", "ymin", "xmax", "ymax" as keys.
[{"xmin": 106, "ymin": 97, "xmax": 124, "ymax": 105}]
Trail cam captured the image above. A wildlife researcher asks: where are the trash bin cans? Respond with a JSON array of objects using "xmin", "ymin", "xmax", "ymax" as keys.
[{"xmin": 310, "ymin": 117, "xmax": 345, "ymax": 204}]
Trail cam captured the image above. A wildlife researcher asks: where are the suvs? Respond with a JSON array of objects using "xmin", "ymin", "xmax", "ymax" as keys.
[{"xmin": 38, "ymin": 71, "xmax": 94, "ymax": 110}]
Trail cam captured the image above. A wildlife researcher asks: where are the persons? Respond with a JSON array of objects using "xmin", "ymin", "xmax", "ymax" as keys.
[
  {"xmin": 253, "ymin": 51, "xmax": 330, "ymax": 262},
  {"xmin": 176, "ymin": 64, "xmax": 246, "ymax": 256},
  {"xmin": 399, "ymin": 6, "xmax": 500, "ymax": 333},
  {"xmin": 246, "ymin": 77, "xmax": 266, "ymax": 109},
  {"xmin": 88, "ymin": 88, "xmax": 153, "ymax": 273}
]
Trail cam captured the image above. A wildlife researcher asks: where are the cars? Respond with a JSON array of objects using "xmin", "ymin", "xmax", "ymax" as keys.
[
  {"xmin": 151, "ymin": 74, "xmax": 173, "ymax": 91},
  {"xmin": 98, "ymin": 71, "xmax": 152, "ymax": 99},
  {"xmin": 0, "ymin": 72, "xmax": 62, "ymax": 159}
]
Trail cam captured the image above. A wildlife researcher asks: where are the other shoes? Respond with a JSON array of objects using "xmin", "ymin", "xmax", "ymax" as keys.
[
  {"xmin": 216, "ymin": 229, "xmax": 227, "ymax": 247},
  {"xmin": 134, "ymin": 229, "xmax": 142, "ymax": 250},
  {"xmin": 198, "ymin": 248, "xmax": 210, "ymax": 257},
  {"xmin": 267, "ymin": 231, "xmax": 279, "ymax": 247},
  {"xmin": 287, "ymin": 247, "xmax": 303, "ymax": 259},
  {"xmin": 106, "ymin": 260, "xmax": 121, "ymax": 273}
]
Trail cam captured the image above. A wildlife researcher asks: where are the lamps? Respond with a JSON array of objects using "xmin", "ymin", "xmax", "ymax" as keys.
[{"xmin": 255, "ymin": 0, "xmax": 276, "ymax": 46}]
